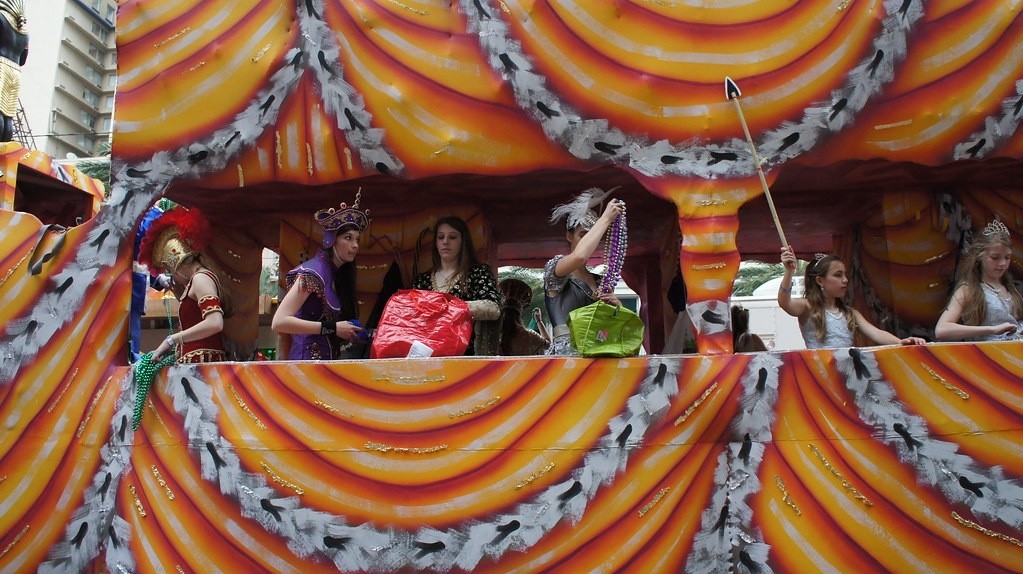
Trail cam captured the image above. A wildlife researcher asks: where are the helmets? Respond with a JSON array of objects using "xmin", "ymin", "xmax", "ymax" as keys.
[{"xmin": 152, "ymin": 226, "xmax": 197, "ymax": 277}]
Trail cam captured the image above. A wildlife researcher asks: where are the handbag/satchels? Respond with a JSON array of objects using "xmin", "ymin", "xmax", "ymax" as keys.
[
  {"xmin": 369, "ymin": 289, "xmax": 473, "ymax": 357},
  {"xmin": 565, "ymin": 301, "xmax": 645, "ymax": 357}
]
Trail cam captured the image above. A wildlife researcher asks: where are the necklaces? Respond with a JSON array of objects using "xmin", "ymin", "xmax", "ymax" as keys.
[
  {"xmin": 132, "ymin": 349, "xmax": 178, "ymax": 431},
  {"xmin": 597, "ymin": 199, "xmax": 628, "ymax": 305},
  {"xmin": 982, "ymin": 280, "xmax": 1000, "ymax": 292},
  {"xmin": 438, "ymin": 269, "xmax": 461, "ymax": 282},
  {"xmin": 826, "ymin": 307, "xmax": 839, "ymax": 314},
  {"xmin": 166, "ymin": 285, "xmax": 174, "ymax": 335}
]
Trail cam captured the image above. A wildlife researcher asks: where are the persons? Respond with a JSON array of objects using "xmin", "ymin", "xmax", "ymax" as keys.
[
  {"xmin": 778, "ymin": 245, "xmax": 927, "ymax": 346},
  {"xmin": 129, "ymin": 205, "xmax": 174, "ymax": 357},
  {"xmin": 934, "ymin": 223, "xmax": 1023, "ymax": 341},
  {"xmin": 497, "ymin": 278, "xmax": 552, "ymax": 355},
  {"xmin": 271, "ymin": 201, "xmax": 372, "ymax": 359},
  {"xmin": 409, "ymin": 215, "xmax": 502, "ymax": 357},
  {"xmin": 144, "ymin": 212, "xmax": 230, "ymax": 363},
  {"xmin": 731, "ymin": 302, "xmax": 768, "ymax": 352},
  {"xmin": 541, "ymin": 197, "xmax": 625, "ymax": 356}
]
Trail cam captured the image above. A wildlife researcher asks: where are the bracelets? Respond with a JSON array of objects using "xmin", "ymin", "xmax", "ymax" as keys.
[
  {"xmin": 780, "ymin": 282, "xmax": 793, "ymax": 293},
  {"xmin": 319, "ymin": 320, "xmax": 336, "ymax": 338},
  {"xmin": 166, "ymin": 335, "xmax": 174, "ymax": 346},
  {"xmin": 536, "ymin": 319, "xmax": 543, "ymax": 323}
]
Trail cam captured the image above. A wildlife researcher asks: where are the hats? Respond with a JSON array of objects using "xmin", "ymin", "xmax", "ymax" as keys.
[
  {"xmin": 314, "ymin": 186, "xmax": 373, "ymax": 249},
  {"xmin": 497, "ymin": 279, "xmax": 532, "ymax": 316}
]
